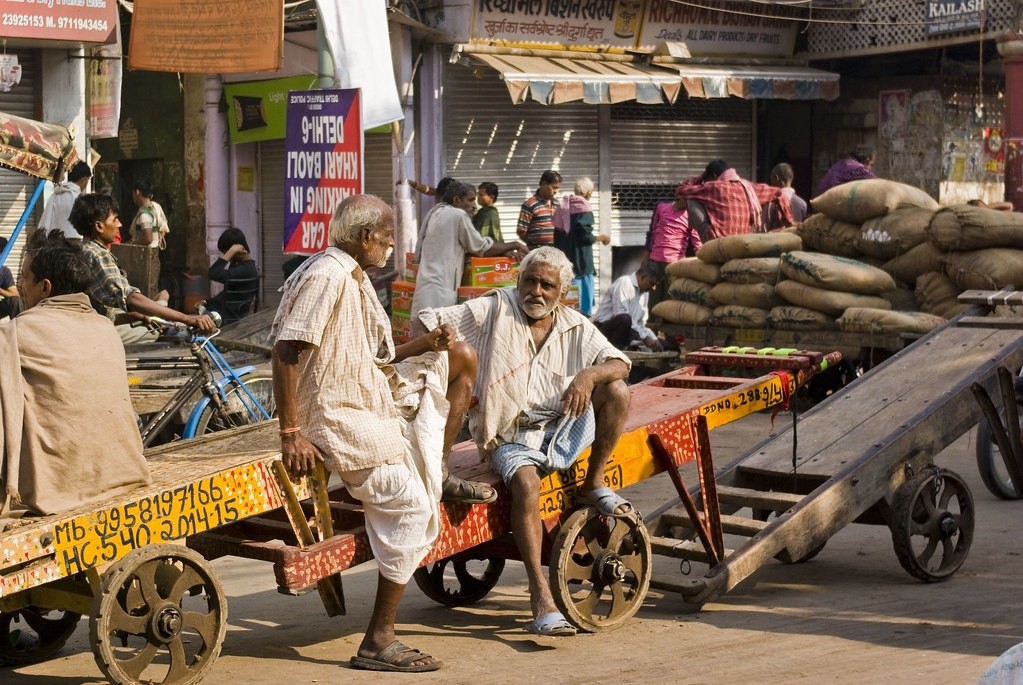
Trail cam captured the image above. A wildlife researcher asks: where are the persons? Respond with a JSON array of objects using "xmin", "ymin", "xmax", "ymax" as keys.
[
  {"xmin": 594, "ymin": 261, "xmax": 664, "ymax": 353},
  {"xmin": 68, "ymin": 192, "xmax": 216, "ymax": 343},
  {"xmin": 194, "ymin": 228, "xmax": 258, "ymax": 328},
  {"xmin": 37, "ymin": 160, "xmax": 92, "ymax": 241},
  {"xmin": 412, "ymin": 247, "xmax": 634, "ymax": 635},
  {"xmin": 0, "ymin": 266, "xmax": 21, "ymax": 320},
  {"xmin": 267, "ymin": 194, "xmax": 498, "ymax": 673},
  {"xmin": 0, "ymin": 228, "xmax": 152, "ymax": 530},
  {"xmin": 554, "ymin": 178, "xmax": 610, "ymax": 318},
  {"xmin": 396, "ymin": 178, "xmax": 504, "ymax": 243},
  {"xmin": 644, "ymin": 161, "xmax": 807, "ymax": 323},
  {"xmin": 124, "ymin": 179, "xmax": 170, "ymax": 250},
  {"xmin": 410, "ymin": 183, "xmax": 529, "ymax": 341},
  {"xmin": 516, "ymin": 170, "xmax": 562, "ymax": 251},
  {"xmin": 813, "ymin": 148, "xmax": 876, "ymax": 200}
]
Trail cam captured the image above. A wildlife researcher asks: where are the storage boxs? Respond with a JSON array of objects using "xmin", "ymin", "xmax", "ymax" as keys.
[{"xmin": 391, "ymin": 255, "xmax": 580, "ymax": 346}]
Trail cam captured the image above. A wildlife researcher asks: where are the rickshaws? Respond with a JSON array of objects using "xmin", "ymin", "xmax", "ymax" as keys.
[
  {"xmin": 0, "ymin": 113, "xmax": 280, "ymax": 440},
  {"xmin": 0, "ymin": 264, "xmax": 844, "ymax": 685},
  {"xmin": 567, "ymin": 281, "xmax": 1022, "ymax": 609}
]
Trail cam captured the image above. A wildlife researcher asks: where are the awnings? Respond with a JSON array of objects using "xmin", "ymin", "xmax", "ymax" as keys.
[
  {"xmin": 454, "ymin": 44, "xmax": 682, "ymax": 105},
  {"xmin": 651, "ymin": 55, "xmax": 840, "ymax": 101}
]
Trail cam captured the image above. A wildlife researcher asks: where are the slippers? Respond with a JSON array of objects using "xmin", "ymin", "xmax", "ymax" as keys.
[
  {"xmin": 441, "ymin": 472, "xmax": 498, "ymax": 503},
  {"xmin": 523, "ymin": 613, "xmax": 578, "ymax": 637},
  {"xmin": 349, "ymin": 640, "xmax": 443, "ymax": 672},
  {"xmin": 576, "ymin": 487, "xmax": 634, "ymax": 517}
]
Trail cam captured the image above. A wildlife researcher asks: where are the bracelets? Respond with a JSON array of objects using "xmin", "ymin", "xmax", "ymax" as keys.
[
  {"xmin": 424, "ymin": 185, "xmax": 429, "ymax": 194},
  {"xmin": 279, "ymin": 426, "xmax": 301, "ymax": 435},
  {"xmin": 203, "ymin": 300, "xmax": 207, "ymax": 304}
]
